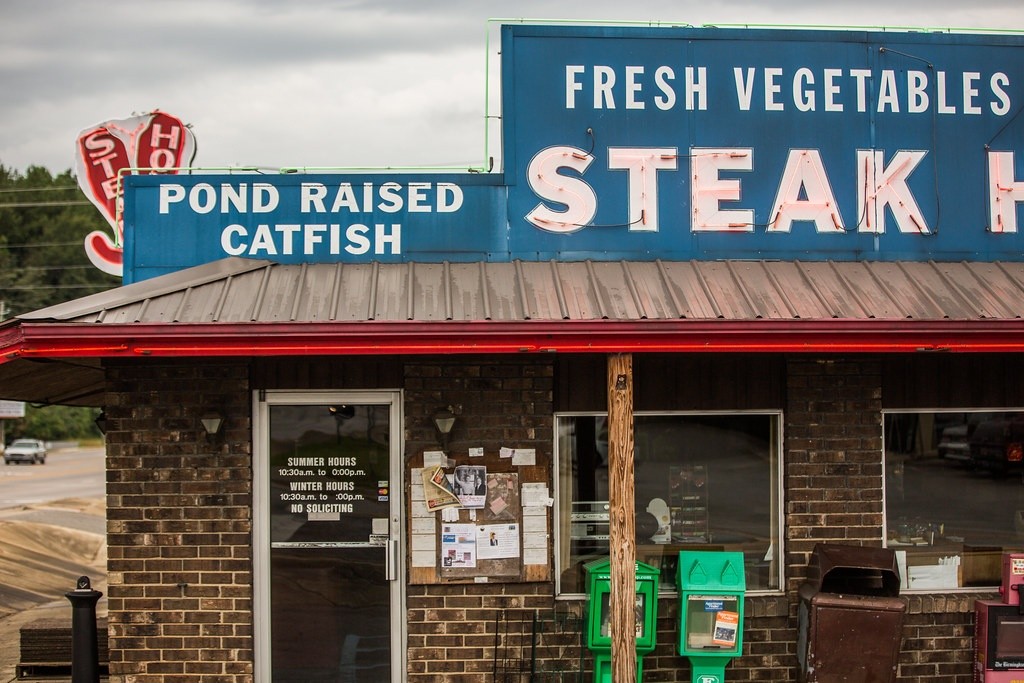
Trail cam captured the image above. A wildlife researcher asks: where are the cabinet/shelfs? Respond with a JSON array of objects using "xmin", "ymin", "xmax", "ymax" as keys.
[{"xmin": 669, "ymin": 459, "xmax": 711, "ymax": 544}]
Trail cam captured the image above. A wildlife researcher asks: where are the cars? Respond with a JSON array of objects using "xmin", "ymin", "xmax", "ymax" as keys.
[
  {"xmin": 4, "ymin": 439, "xmax": 47, "ymax": 465},
  {"xmin": 938, "ymin": 411, "xmax": 1023, "ymax": 486},
  {"xmin": 270, "ymin": 466, "xmax": 312, "ymax": 515}
]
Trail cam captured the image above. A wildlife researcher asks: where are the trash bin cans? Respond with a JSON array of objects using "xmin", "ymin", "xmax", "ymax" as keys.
[{"xmin": 796, "ymin": 543, "xmax": 906, "ymax": 683}]
